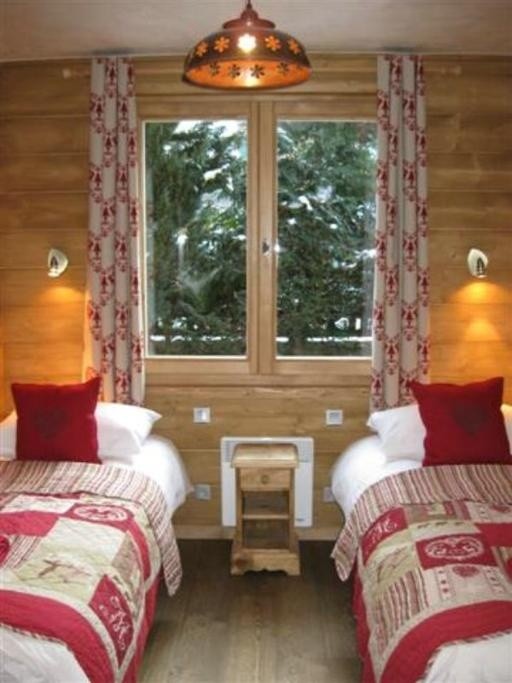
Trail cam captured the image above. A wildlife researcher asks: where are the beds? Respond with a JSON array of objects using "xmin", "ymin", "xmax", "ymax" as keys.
[
  {"xmin": 329, "ymin": 374, "xmax": 512, "ymax": 683},
  {"xmin": 0, "ymin": 372, "xmax": 197, "ymax": 683}
]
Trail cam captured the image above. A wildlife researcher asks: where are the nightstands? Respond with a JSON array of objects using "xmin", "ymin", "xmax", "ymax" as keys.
[{"xmin": 229, "ymin": 443, "xmax": 301, "ymax": 576}]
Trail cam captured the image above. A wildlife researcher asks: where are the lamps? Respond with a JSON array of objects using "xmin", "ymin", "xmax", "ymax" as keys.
[
  {"xmin": 466, "ymin": 246, "xmax": 489, "ymax": 278},
  {"xmin": 182, "ymin": 0, "xmax": 314, "ymax": 92},
  {"xmin": 43, "ymin": 247, "xmax": 69, "ymax": 276}
]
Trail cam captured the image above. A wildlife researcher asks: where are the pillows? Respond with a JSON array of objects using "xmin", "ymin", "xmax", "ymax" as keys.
[
  {"xmin": 364, "ymin": 398, "xmax": 512, "ymax": 466},
  {"xmin": 11, "ymin": 375, "xmax": 105, "ymax": 464},
  {"xmin": 404, "ymin": 377, "xmax": 511, "ymax": 465},
  {"xmin": 1, "ymin": 400, "xmax": 163, "ymax": 464}
]
